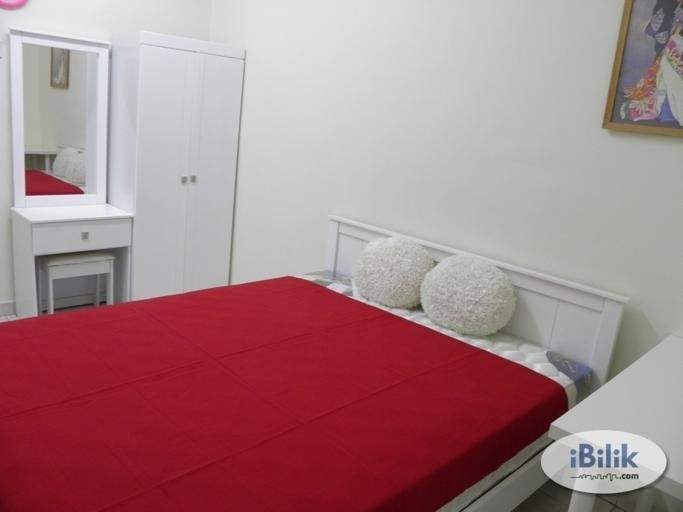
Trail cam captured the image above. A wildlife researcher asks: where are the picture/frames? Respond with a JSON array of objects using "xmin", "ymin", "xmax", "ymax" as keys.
[
  {"xmin": 50, "ymin": 46, "xmax": 70, "ymax": 90},
  {"xmin": 601, "ymin": 1, "xmax": 683, "ymax": 137}
]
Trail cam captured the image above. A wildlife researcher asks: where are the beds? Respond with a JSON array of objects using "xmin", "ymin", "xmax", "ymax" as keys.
[
  {"xmin": 1, "ymin": 214, "xmax": 631, "ymax": 510},
  {"xmin": 25, "ymin": 147, "xmax": 86, "ymax": 195}
]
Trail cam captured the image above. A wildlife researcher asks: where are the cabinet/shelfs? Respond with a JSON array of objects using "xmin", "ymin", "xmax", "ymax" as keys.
[
  {"xmin": 112, "ymin": 30, "xmax": 247, "ymax": 300},
  {"xmin": 10, "ymin": 206, "xmax": 135, "ymax": 321}
]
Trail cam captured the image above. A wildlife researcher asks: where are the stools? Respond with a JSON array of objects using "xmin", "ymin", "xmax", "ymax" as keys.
[{"xmin": 41, "ymin": 254, "xmax": 118, "ymax": 314}]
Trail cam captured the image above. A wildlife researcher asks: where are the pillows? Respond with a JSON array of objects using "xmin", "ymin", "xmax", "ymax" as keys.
[
  {"xmin": 53, "ymin": 149, "xmax": 85, "ymax": 183},
  {"xmin": 352, "ymin": 234, "xmax": 518, "ymax": 336}
]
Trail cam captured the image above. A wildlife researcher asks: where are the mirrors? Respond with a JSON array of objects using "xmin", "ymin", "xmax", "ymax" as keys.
[{"xmin": 11, "ymin": 28, "xmax": 111, "ymax": 204}]
